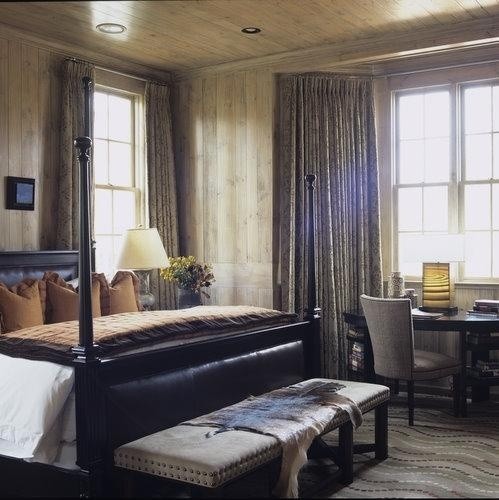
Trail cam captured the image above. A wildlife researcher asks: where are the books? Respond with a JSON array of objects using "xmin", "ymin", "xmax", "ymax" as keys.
[
  {"xmin": 467, "ymin": 300, "xmax": 499, "ymax": 314},
  {"xmin": 349, "ymin": 342, "xmax": 366, "ymax": 372},
  {"xmin": 467, "ymin": 331, "xmax": 499, "ymax": 378}
]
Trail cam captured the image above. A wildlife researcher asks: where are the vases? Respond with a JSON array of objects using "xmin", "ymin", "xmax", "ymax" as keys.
[{"xmin": 179, "ymin": 287, "xmax": 203, "ymax": 310}]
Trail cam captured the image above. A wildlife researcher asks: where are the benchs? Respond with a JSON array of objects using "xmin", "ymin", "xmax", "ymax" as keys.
[{"xmin": 108, "ymin": 378, "xmax": 391, "ymax": 498}]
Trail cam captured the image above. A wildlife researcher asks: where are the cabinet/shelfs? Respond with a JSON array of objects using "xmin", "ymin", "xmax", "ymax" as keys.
[{"xmin": 343, "ymin": 307, "xmax": 499, "ymax": 421}]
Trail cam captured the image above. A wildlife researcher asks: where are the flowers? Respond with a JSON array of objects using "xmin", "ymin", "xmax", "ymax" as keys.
[{"xmin": 160, "ymin": 257, "xmax": 214, "ymax": 299}]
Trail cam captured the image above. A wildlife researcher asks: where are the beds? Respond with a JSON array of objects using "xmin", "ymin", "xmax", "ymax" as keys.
[{"xmin": 0, "ymin": 250, "xmax": 317, "ymax": 500}]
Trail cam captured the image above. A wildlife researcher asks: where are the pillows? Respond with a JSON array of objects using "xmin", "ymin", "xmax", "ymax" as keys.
[{"xmin": 0, "ymin": 270, "xmax": 140, "ymax": 335}]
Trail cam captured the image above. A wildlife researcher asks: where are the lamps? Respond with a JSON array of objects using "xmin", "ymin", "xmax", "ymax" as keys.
[
  {"xmin": 418, "ymin": 261, "xmax": 458, "ymax": 315},
  {"xmin": 117, "ymin": 228, "xmax": 170, "ymax": 311}
]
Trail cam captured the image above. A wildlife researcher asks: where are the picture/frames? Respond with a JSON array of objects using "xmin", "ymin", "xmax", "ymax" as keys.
[{"xmin": 6, "ymin": 176, "xmax": 35, "ymax": 211}]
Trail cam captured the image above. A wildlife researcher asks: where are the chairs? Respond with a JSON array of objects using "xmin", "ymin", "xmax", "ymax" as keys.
[{"xmin": 359, "ymin": 292, "xmax": 462, "ymax": 425}]
